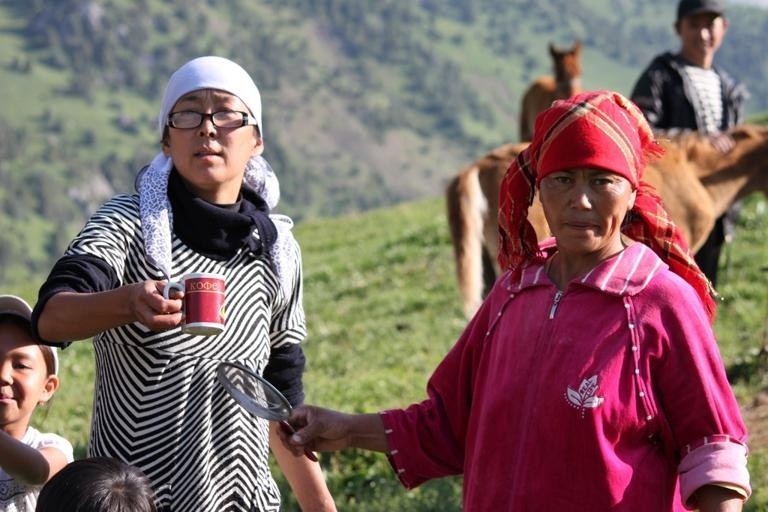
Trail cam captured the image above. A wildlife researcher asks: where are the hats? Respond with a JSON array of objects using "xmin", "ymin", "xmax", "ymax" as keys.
[
  {"xmin": 677, "ymin": 0, "xmax": 725, "ymax": 16},
  {"xmin": 160, "ymin": 56, "xmax": 262, "ymax": 138},
  {"xmin": 0, "ymin": 295, "xmax": 59, "ymax": 376}
]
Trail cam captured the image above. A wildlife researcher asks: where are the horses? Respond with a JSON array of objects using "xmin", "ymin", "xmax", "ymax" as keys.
[
  {"xmin": 447, "ymin": 123, "xmax": 767, "ymax": 324},
  {"xmin": 517, "ymin": 38, "xmax": 584, "ymax": 141}
]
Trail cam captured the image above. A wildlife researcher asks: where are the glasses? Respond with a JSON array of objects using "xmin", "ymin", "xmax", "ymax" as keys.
[{"xmin": 167, "ymin": 111, "xmax": 257, "ymax": 129}]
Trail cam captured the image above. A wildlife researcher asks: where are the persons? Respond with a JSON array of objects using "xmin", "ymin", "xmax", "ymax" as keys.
[
  {"xmin": 29, "ymin": 54, "xmax": 339, "ymax": 512},
  {"xmin": 34, "ymin": 457, "xmax": 159, "ymax": 512},
  {"xmin": 277, "ymin": 92, "xmax": 752, "ymax": 511},
  {"xmin": 1, "ymin": 293, "xmax": 74, "ymax": 512},
  {"xmin": 630, "ymin": 0, "xmax": 746, "ymax": 295}
]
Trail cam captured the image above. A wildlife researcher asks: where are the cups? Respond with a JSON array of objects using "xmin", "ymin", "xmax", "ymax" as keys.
[{"xmin": 162, "ymin": 274, "xmax": 227, "ymax": 337}]
